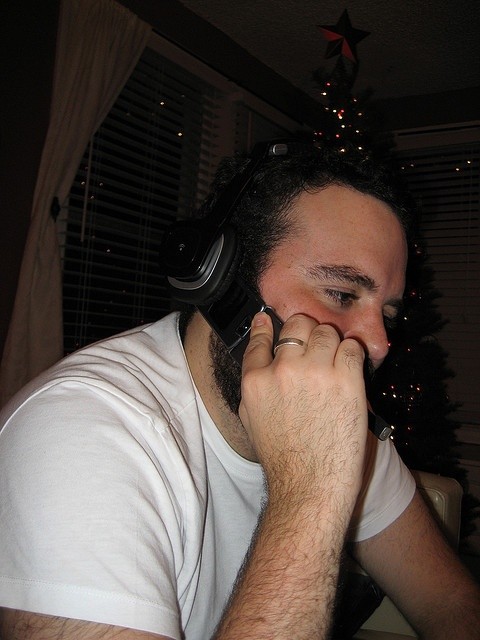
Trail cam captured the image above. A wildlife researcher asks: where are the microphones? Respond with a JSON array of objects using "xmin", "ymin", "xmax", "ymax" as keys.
[{"xmin": 367, "ymin": 408, "xmax": 396, "ymax": 442}]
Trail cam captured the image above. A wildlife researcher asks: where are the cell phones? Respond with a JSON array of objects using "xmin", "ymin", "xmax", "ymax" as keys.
[{"xmin": 198, "ymin": 278, "xmax": 285, "ymax": 375}]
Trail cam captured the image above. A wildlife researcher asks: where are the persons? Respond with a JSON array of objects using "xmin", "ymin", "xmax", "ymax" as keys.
[{"xmin": 1, "ymin": 137, "xmax": 479, "ymax": 640}]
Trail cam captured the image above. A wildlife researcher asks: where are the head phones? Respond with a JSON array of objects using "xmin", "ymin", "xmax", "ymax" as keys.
[{"xmin": 158, "ymin": 140, "xmax": 291, "ymax": 306}]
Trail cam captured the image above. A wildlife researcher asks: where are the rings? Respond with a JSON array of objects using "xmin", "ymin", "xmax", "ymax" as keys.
[{"xmin": 274, "ymin": 339, "xmax": 306, "ymax": 350}]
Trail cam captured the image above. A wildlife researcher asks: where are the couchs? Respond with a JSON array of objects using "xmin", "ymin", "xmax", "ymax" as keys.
[{"xmin": 349, "ymin": 469, "xmax": 463, "ymax": 638}]
